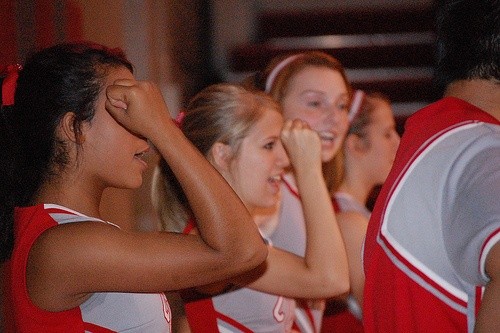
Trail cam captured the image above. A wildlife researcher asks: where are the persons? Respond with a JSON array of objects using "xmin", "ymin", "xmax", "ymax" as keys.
[
  {"xmin": 149, "ymin": 83, "xmax": 350, "ymax": 333},
  {"xmin": 322, "ymin": 90, "xmax": 400, "ymax": 333},
  {"xmin": 363, "ymin": 1, "xmax": 500, "ymax": 333},
  {"xmin": 0, "ymin": 42, "xmax": 268, "ymax": 333},
  {"xmin": 266, "ymin": 53, "xmax": 350, "ymax": 332}
]
